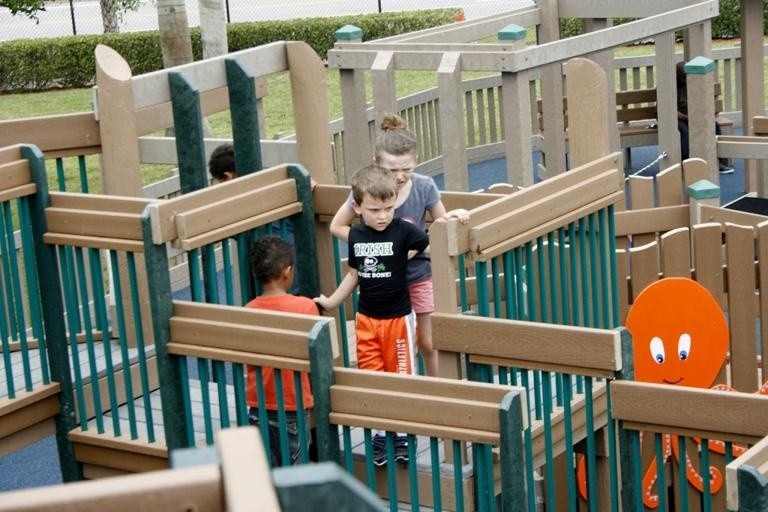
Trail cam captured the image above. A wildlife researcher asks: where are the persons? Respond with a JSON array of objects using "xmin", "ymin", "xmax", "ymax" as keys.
[
  {"xmin": 208, "ymin": 142, "xmax": 301, "ymax": 296},
  {"xmin": 312, "ymin": 165, "xmax": 429, "ymax": 466},
  {"xmin": 244, "ymin": 236, "xmax": 321, "ymax": 465},
  {"xmin": 328, "ymin": 113, "xmax": 471, "ymax": 378}
]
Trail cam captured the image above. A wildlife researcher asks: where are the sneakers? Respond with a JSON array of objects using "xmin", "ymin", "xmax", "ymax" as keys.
[
  {"xmin": 368, "ymin": 433, "xmax": 388, "ymax": 467},
  {"xmin": 392, "ymin": 434, "xmax": 415, "ymax": 465}
]
